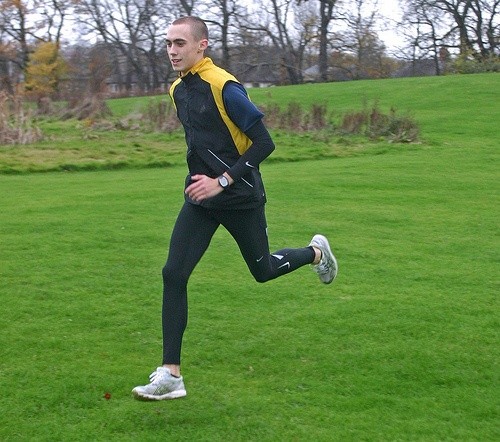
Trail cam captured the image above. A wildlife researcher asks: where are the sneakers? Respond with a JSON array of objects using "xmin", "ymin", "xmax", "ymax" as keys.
[
  {"xmin": 132, "ymin": 367, "xmax": 186, "ymax": 402},
  {"xmin": 308, "ymin": 234, "xmax": 338, "ymax": 285}
]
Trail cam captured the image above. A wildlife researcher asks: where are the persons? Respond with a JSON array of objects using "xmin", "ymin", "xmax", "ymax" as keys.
[{"xmin": 130, "ymin": 16, "xmax": 338, "ymax": 403}]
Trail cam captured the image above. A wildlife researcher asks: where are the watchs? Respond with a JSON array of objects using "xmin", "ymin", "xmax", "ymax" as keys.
[{"xmin": 218, "ymin": 174, "xmax": 229, "ymax": 190}]
